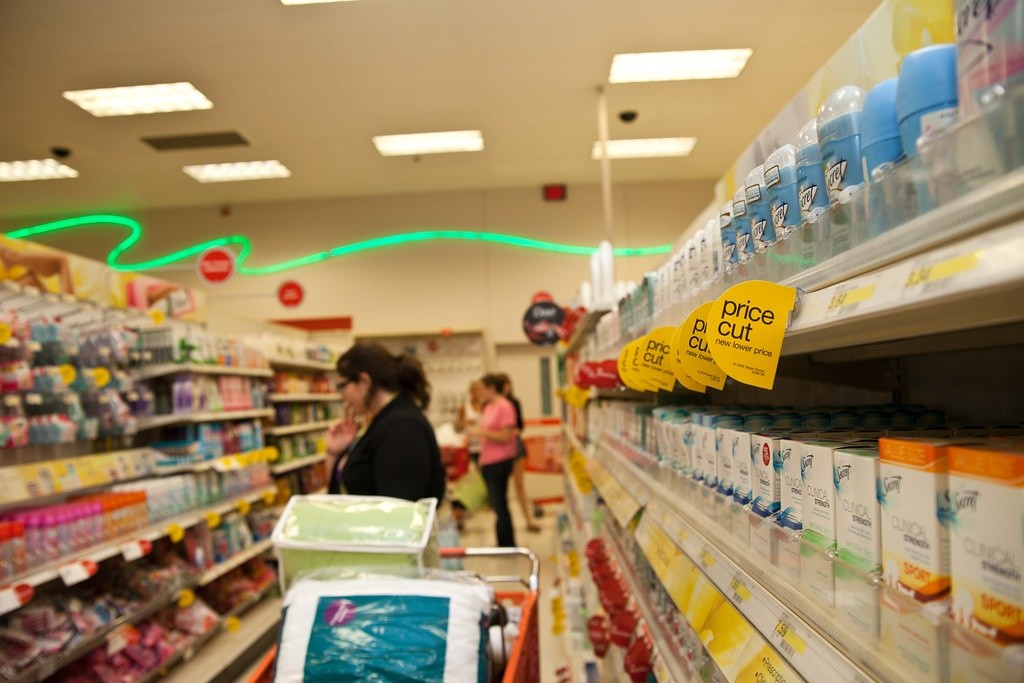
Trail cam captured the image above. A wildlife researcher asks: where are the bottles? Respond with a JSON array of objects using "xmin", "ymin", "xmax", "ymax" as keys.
[
  {"xmin": 861, "ymin": 77, "xmax": 909, "ymax": 242},
  {"xmin": 720, "ymin": 85, "xmax": 864, "ymax": 292},
  {"xmin": 895, "ymin": 43, "xmax": 958, "ymax": 222}
]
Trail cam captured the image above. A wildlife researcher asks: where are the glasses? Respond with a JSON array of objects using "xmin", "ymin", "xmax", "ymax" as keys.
[{"xmin": 336, "ymin": 373, "xmax": 358, "ymax": 391}]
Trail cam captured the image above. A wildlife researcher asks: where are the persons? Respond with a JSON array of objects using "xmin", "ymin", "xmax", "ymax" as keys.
[
  {"xmin": 454, "ymin": 373, "xmax": 540, "ymax": 548},
  {"xmin": 322, "ymin": 343, "xmax": 445, "ymax": 504}
]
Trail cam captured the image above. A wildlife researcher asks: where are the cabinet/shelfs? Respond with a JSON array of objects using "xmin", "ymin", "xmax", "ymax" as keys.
[
  {"xmin": 559, "ymin": 86, "xmax": 1024, "ymax": 683},
  {"xmin": 0, "ymin": 361, "xmax": 346, "ymax": 682}
]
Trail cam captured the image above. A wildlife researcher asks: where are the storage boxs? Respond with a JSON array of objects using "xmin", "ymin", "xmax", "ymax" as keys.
[
  {"xmin": 272, "ymin": 493, "xmax": 440, "ymax": 598},
  {"xmin": 596, "ymin": 395, "xmax": 1024, "ymax": 654}
]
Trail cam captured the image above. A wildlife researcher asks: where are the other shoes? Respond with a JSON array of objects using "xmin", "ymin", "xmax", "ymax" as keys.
[{"xmin": 527, "ymin": 524, "xmax": 541, "ymax": 533}]
